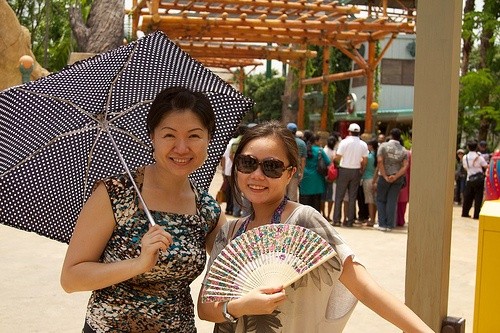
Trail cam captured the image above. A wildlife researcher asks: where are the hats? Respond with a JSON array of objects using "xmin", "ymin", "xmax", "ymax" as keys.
[
  {"xmin": 247, "ymin": 123, "xmax": 258, "ymax": 128},
  {"xmin": 479, "ymin": 141, "xmax": 487, "ymax": 146},
  {"xmin": 347, "ymin": 123, "xmax": 360, "ymax": 132},
  {"xmin": 286, "ymin": 123, "xmax": 297, "ymax": 132},
  {"xmin": 456, "ymin": 149, "xmax": 464, "ymax": 154}
]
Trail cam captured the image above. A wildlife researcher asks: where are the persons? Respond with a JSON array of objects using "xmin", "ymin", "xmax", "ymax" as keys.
[
  {"xmin": 221, "ymin": 121, "xmax": 412, "ymax": 232},
  {"xmin": 453, "ymin": 139, "xmax": 500, "ymax": 219},
  {"xmin": 197, "ymin": 119, "xmax": 435, "ymax": 333},
  {"xmin": 60, "ymin": 86, "xmax": 227, "ymax": 333}
]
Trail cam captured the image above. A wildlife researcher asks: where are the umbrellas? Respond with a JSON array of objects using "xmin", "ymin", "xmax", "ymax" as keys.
[{"xmin": 0, "ymin": 30, "xmax": 255, "ymax": 246}]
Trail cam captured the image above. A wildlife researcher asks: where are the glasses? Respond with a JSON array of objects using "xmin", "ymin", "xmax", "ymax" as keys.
[{"xmin": 235, "ymin": 154, "xmax": 292, "ymax": 179}]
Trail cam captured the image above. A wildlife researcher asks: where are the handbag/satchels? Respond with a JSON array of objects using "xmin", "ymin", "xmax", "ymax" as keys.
[
  {"xmin": 469, "ymin": 172, "xmax": 485, "ymax": 186},
  {"xmin": 327, "ymin": 159, "xmax": 338, "ymax": 181},
  {"xmin": 317, "ymin": 147, "xmax": 327, "ymax": 174}
]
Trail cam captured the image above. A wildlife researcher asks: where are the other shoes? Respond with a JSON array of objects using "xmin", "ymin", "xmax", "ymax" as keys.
[
  {"xmin": 373, "ymin": 224, "xmax": 390, "ymax": 232},
  {"xmin": 323, "ymin": 217, "xmax": 375, "ymax": 227}
]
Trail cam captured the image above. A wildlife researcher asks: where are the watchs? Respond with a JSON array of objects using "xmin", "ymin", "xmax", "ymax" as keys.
[{"xmin": 222, "ymin": 302, "xmax": 237, "ymax": 324}]
